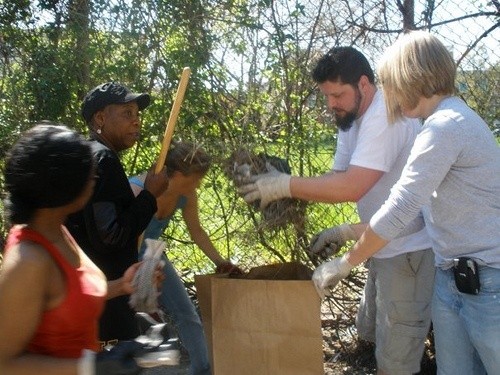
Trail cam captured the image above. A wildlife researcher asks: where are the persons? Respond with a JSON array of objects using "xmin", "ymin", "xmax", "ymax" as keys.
[
  {"xmin": 0, "ymin": 123, "xmax": 163, "ymax": 375},
  {"xmin": 240, "ymin": 47, "xmax": 436, "ymax": 375},
  {"xmin": 63, "ymin": 82, "xmax": 169, "ymax": 375},
  {"xmin": 310, "ymin": 28, "xmax": 500, "ymax": 375},
  {"xmin": 128, "ymin": 140, "xmax": 239, "ymax": 375}
]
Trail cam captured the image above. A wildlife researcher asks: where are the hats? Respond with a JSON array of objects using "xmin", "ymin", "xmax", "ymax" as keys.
[
  {"xmin": 80, "ymin": 82, "xmax": 150, "ymax": 123},
  {"xmin": 77, "ymin": 342, "xmax": 145, "ymax": 375}
]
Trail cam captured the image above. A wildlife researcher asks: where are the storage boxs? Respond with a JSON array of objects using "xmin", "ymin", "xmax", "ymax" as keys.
[{"xmin": 194, "ymin": 260, "xmax": 324, "ymax": 375}]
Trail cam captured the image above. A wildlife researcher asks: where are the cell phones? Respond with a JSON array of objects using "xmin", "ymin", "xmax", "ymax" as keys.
[{"xmin": 452, "ymin": 259, "xmax": 478, "ymax": 294}]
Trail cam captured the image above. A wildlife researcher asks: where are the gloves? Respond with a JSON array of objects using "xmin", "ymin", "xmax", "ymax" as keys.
[
  {"xmin": 311, "ymin": 253, "xmax": 356, "ymax": 299},
  {"xmin": 309, "ymin": 224, "xmax": 357, "ymax": 258},
  {"xmin": 216, "ymin": 262, "xmax": 243, "ymax": 277},
  {"xmin": 237, "ymin": 162, "xmax": 292, "ymax": 210}
]
